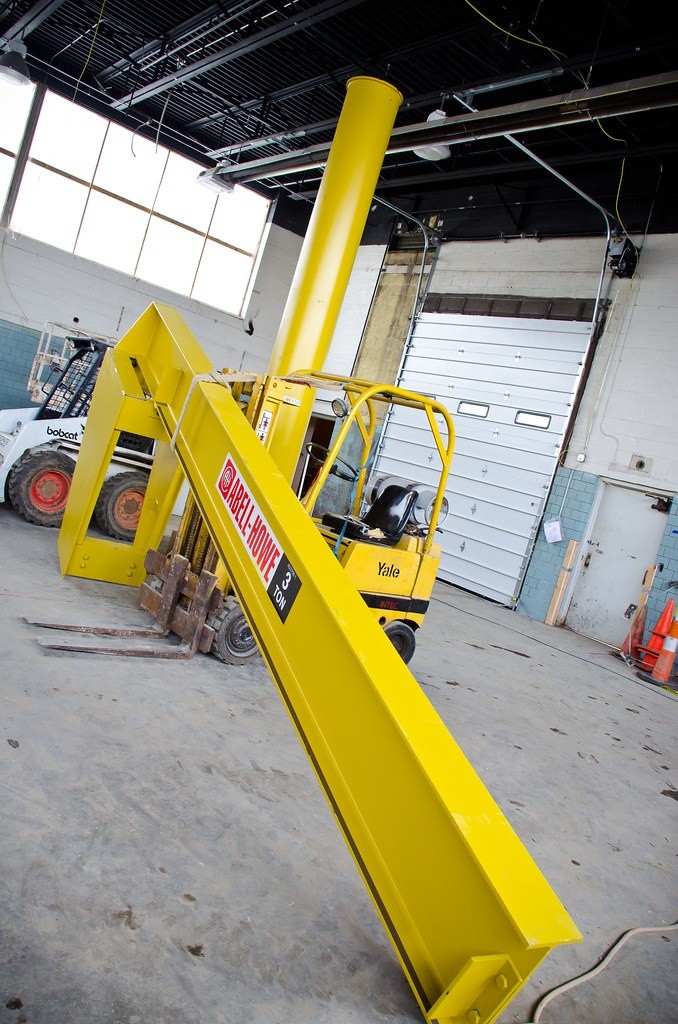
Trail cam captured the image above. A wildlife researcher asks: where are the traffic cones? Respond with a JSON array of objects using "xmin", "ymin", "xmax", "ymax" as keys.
[
  {"xmin": 633, "ymin": 597, "xmax": 678, "ymax": 687},
  {"xmin": 609, "ymin": 603, "xmax": 647, "ymax": 661}
]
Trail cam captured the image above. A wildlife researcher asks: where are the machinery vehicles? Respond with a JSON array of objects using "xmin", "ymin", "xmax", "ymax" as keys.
[
  {"xmin": 0, "ymin": 335, "xmax": 160, "ymax": 538},
  {"xmin": 17, "ymin": 365, "xmax": 455, "ymax": 676}
]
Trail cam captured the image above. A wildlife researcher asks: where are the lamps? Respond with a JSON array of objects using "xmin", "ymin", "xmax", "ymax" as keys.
[
  {"xmin": 413, "ymin": 92, "xmax": 453, "ymax": 161},
  {"xmin": 0, "ymin": 26, "xmax": 31, "ymax": 87}
]
